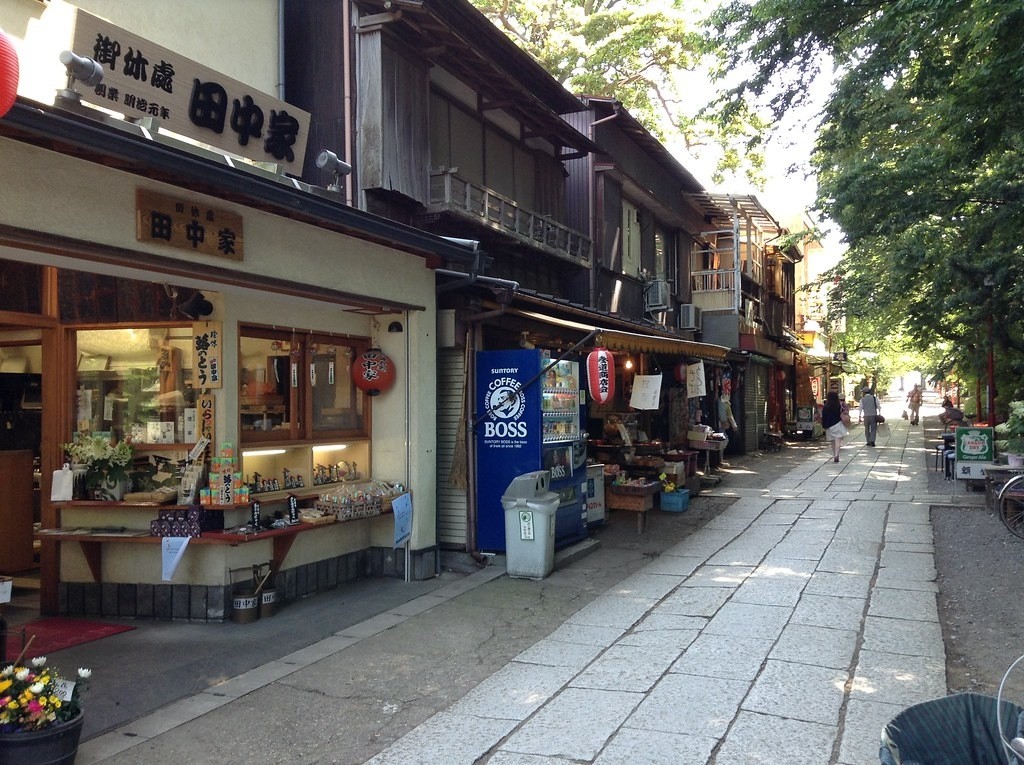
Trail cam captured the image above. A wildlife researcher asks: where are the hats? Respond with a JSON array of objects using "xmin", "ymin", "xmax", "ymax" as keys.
[{"xmin": 862, "ymin": 387, "xmax": 869, "ymax": 392}]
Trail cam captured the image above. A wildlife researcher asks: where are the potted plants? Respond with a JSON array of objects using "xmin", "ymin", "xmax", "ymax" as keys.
[{"xmin": 994, "ymin": 438, "xmax": 1024, "ymax": 468}]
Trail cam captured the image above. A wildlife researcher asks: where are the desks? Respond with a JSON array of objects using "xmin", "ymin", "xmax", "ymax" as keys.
[{"xmin": 940, "ymin": 433, "xmax": 956, "ymax": 475}]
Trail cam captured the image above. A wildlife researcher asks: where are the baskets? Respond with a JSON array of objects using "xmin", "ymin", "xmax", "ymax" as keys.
[
  {"xmin": 298, "ymin": 515, "xmax": 337, "ymax": 526},
  {"xmin": 313, "ymin": 496, "xmax": 381, "ymax": 519},
  {"xmin": 382, "ymin": 489, "xmax": 411, "ymax": 512}
]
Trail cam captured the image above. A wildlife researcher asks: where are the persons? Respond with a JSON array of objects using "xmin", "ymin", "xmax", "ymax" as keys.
[
  {"xmin": 858, "ymin": 386, "xmax": 881, "ymax": 446},
  {"xmin": 822, "ymin": 391, "xmax": 848, "ymax": 462},
  {"xmin": 939, "ymin": 395, "xmax": 953, "ymax": 424},
  {"xmin": 908, "ymin": 383, "xmax": 923, "ymax": 425},
  {"xmin": 718, "ymin": 386, "xmax": 738, "ymax": 461},
  {"xmin": 838, "ymin": 393, "xmax": 851, "ymax": 423}
]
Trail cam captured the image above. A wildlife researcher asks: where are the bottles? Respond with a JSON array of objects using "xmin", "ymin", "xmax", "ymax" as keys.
[{"xmin": 549, "ymin": 371, "xmax": 556, "ymax": 387}]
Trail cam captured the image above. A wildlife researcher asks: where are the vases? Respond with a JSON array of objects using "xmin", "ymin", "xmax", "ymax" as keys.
[
  {"xmin": 0, "ymin": 706, "xmax": 84, "ymax": 765},
  {"xmin": 69, "ymin": 464, "xmax": 92, "ymax": 500}
]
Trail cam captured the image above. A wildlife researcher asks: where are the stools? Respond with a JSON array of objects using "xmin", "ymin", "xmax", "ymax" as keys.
[
  {"xmin": 934, "ymin": 442, "xmax": 955, "ymax": 483},
  {"xmin": 762, "ymin": 431, "xmax": 784, "ymax": 454}
]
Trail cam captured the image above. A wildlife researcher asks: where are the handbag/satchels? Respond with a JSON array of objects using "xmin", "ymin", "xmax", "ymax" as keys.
[
  {"xmin": 875, "ymin": 415, "xmax": 885, "ymax": 423},
  {"xmin": 721, "ymin": 422, "xmax": 730, "ymax": 429},
  {"xmin": 840, "ymin": 403, "xmax": 851, "ymax": 427}
]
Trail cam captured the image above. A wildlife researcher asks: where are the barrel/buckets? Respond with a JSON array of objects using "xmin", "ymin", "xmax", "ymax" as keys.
[{"xmin": 228, "ymin": 559, "xmax": 277, "ymax": 624}]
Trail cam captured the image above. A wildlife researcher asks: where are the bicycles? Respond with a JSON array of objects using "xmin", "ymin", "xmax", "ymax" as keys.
[
  {"xmin": 945, "ymin": 407, "xmax": 997, "ymax": 433},
  {"xmin": 996, "ymin": 470, "xmax": 1024, "ymax": 540},
  {"xmin": 879, "ymin": 655, "xmax": 1024, "ymax": 765}
]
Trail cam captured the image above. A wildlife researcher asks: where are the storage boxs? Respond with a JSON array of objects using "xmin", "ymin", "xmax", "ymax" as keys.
[
  {"xmin": 687, "ymin": 432, "xmax": 708, "ymax": 442},
  {"xmin": 199, "ymin": 443, "xmax": 249, "ymax": 505},
  {"xmin": 658, "ymin": 466, "xmax": 684, "ymax": 473},
  {"xmin": 660, "ymin": 488, "xmax": 691, "ymax": 512}
]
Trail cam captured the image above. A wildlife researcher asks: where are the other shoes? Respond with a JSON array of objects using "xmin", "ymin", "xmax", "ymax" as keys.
[
  {"xmin": 866, "ymin": 441, "xmax": 876, "ymax": 446},
  {"xmin": 911, "ymin": 421, "xmax": 918, "ymax": 425},
  {"xmin": 834, "ymin": 456, "xmax": 839, "ymax": 462}
]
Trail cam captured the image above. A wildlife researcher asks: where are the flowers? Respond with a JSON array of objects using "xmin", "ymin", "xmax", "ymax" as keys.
[
  {"xmin": 57, "ymin": 434, "xmax": 132, "ymax": 481},
  {"xmin": 0, "ymin": 656, "xmax": 92, "ymax": 732}
]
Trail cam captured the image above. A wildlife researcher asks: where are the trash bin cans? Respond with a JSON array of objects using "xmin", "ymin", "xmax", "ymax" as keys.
[
  {"xmin": 500, "ymin": 470, "xmax": 560, "ymax": 579},
  {"xmin": 880, "ymin": 692, "xmax": 1024, "ymax": 765}
]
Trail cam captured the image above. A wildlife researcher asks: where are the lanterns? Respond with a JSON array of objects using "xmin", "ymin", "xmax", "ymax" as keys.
[
  {"xmin": 674, "ymin": 362, "xmax": 687, "ymax": 385},
  {"xmin": 352, "ymin": 348, "xmax": 395, "ymax": 396},
  {"xmin": 587, "ymin": 347, "xmax": 615, "ymax": 404},
  {"xmin": 0, "ymin": 28, "xmax": 20, "ymax": 117}
]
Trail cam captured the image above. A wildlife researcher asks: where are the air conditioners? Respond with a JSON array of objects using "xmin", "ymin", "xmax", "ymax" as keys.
[
  {"xmin": 681, "ymin": 304, "xmax": 701, "ymax": 331},
  {"xmin": 648, "ymin": 278, "xmax": 671, "ymax": 309}
]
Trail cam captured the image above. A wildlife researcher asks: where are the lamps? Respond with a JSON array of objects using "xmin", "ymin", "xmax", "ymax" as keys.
[
  {"xmin": 54, "ymin": 49, "xmax": 104, "ymax": 102},
  {"xmin": 316, "ymin": 148, "xmax": 353, "ymax": 195}
]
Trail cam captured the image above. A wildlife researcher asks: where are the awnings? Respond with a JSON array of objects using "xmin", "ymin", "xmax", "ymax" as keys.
[{"xmin": 475, "ymin": 300, "xmax": 731, "ymax": 425}]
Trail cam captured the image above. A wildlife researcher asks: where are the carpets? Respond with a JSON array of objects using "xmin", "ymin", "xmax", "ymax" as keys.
[{"xmin": 5, "ymin": 617, "xmax": 138, "ymax": 663}]
